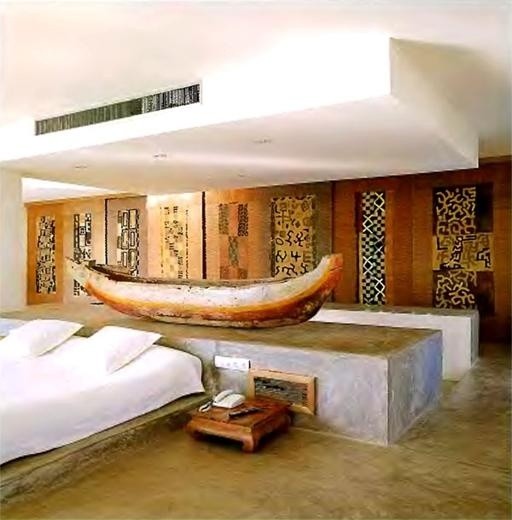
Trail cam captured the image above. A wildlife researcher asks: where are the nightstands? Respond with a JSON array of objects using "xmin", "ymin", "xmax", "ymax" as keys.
[{"xmin": 186, "ymin": 395, "xmax": 292, "ymax": 452}]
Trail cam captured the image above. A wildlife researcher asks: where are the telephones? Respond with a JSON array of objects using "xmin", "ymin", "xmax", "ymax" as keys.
[{"xmin": 212, "ymin": 389, "xmax": 246, "ymax": 409}]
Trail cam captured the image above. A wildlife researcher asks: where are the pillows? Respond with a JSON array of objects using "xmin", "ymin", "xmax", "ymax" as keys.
[
  {"xmin": 82, "ymin": 326, "xmax": 162, "ymax": 372},
  {"xmin": 8, "ymin": 319, "xmax": 83, "ymax": 356}
]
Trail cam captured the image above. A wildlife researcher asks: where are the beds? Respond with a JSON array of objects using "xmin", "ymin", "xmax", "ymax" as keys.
[{"xmin": 0, "ymin": 322, "xmax": 206, "ymax": 463}]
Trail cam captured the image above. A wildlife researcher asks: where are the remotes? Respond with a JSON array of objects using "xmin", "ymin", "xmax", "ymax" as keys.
[{"xmin": 229, "ymin": 407, "xmax": 262, "ymax": 419}]
